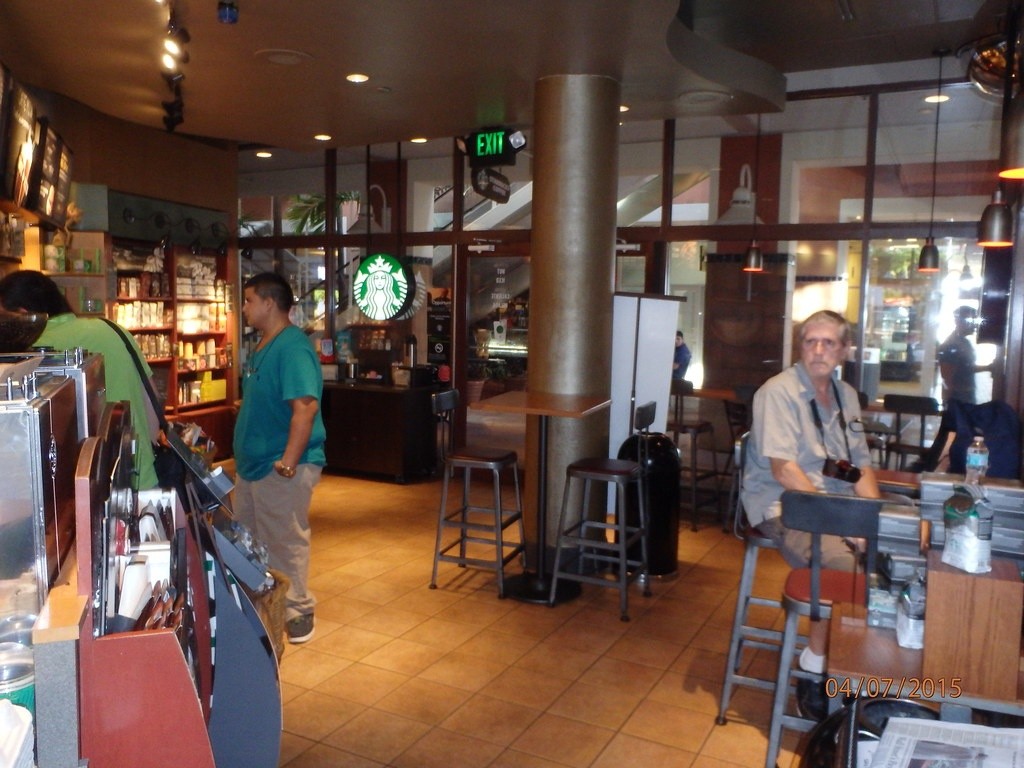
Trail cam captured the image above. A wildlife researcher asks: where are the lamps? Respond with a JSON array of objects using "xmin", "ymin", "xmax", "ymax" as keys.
[
  {"xmin": 979, "ymin": 0, "xmax": 1024, "ymax": 247},
  {"xmin": 122, "ymin": 207, "xmax": 259, "ymax": 260},
  {"xmin": 742, "ymin": 114, "xmax": 764, "ymax": 271},
  {"xmin": 455, "ymin": 124, "xmax": 527, "ymax": 168},
  {"xmin": 918, "ymin": 47, "xmax": 951, "ymax": 270},
  {"xmin": 714, "ymin": 163, "xmax": 764, "ymax": 223},
  {"xmin": 162, "ymin": 21, "xmax": 191, "ymax": 130}
]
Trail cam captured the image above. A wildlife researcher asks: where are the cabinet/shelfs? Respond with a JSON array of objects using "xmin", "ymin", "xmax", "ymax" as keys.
[
  {"xmin": 319, "ymin": 379, "xmax": 436, "ymax": 485},
  {"xmin": 496, "ymin": 301, "xmax": 528, "ymax": 332},
  {"xmin": 0, "ymin": 200, "xmax": 237, "ymax": 464}
]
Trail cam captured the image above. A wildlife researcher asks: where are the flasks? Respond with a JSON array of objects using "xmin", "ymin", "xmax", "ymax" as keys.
[{"xmin": 404, "ymin": 334, "xmax": 417, "ymax": 367}]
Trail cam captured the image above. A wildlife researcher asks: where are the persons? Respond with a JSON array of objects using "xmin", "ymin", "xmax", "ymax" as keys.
[
  {"xmin": 673, "ymin": 330, "xmax": 691, "ymax": 378},
  {"xmin": 740, "ymin": 310, "xmax": 880, "ymax": 723},
  {"xmin": 939, "ymin": 307, "xmax": 995, "ymax": 407},
  {"xmin": 234, "ymin": 274, "xmax": 326, "ymax": 643},
  {"xmin": 0, "ymin": 269, "xmax": 159, "ymax": 491}
]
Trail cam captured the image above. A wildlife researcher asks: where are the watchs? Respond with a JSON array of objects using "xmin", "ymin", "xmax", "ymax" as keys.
[{"xmin": 279, "ymin": 462, "xmax": 296, "ymax": 477}]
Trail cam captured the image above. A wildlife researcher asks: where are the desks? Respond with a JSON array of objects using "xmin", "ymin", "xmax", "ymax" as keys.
[
  {"xmin": 874, "ymin": 467, "xmax": 1024, "ymax": 495},
  {"xmin": 468, "ymin": 390, "xmax": 613, "ymax": 606},
  {"xmin": 689, "ymin": 388, "xmax": 737, "ymax": 400},
  {"xmin": 825, "ymin": 549, "xmax": 1024, "ymax": 768},
  {"xmin": 474, "ymin": 335, "xmax": 528, "ymax": 357}
]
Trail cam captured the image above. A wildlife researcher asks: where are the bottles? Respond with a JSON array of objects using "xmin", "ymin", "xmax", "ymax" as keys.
[{"xmin": 965, "ymin": 435, "xmax": 989, "ymax": 492}]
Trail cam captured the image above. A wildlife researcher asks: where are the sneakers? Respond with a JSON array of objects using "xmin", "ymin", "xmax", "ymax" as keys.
[
  {"xmin": 795, "ymin": 662, "xmax": 828, "ymax": 722},
  {"xmin": 288, "ymin": 610, "xmax": 315, "ymax": 643}
]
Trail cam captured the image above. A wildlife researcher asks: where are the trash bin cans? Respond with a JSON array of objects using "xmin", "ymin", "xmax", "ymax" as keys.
[
  {"xmin": 613, "ymin": 432, "xmax": 681, "ymax": 581},
  {"xmin": 843, "ymin": 346, "xmax": 880, "ymax": 401}
]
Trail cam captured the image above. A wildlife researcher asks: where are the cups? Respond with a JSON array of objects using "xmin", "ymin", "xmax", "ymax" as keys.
[{"xmin": 85, "ymin": 299, "xmax": 94, "ymax": 311}]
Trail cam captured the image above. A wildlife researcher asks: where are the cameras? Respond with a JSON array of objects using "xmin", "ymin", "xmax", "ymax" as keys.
[{"xmin": 822, "ymin": 458, "xmax": 862, "ymax": 483}]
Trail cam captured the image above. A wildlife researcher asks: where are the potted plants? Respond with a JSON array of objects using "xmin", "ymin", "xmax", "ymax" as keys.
[{"xmin": 465, "ymin": 362, "xmax": 528, "ymax": 405}]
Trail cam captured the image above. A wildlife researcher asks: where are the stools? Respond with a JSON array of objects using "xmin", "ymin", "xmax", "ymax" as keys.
[
  {"xmin": 857, "ymin": 391, "xmax": 884, "ymax": 470},
  {"xmin": 716, "ymin": 432, "xmax": 809, "ymax": 726},
  {"xmin": 548, "ymin": 400, "xmax": 657, "ymax": 622},
  {"xmin": 430, "ymin": 390, "xmax": 529, "ymax": 599},
  {"xmin": 718, "ymin": 385, "xmax": 758, "ymax": 533},
  {"xmin": 766, "ymin": 489, "xmax": 914, "ymax": 768},
  {"xmin": 666, "ymin": 378, "xmax": 722, "ymax": 533}
]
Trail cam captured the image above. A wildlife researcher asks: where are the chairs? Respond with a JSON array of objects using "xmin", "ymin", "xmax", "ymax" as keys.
[{"xmin": 884, "ymin": 394, "xmax": 938, "ymax": 471}]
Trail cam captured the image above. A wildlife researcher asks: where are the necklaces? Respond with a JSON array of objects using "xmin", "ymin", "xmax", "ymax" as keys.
[{"xmin": 246, "ymin": 322, "xmax": 291, "ymax": 378}]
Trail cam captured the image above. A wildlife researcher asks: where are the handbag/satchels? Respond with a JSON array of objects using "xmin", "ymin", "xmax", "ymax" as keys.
[{"xmin": 152, "ymin": 450, "xmax": 221, "ymax": 516}]
